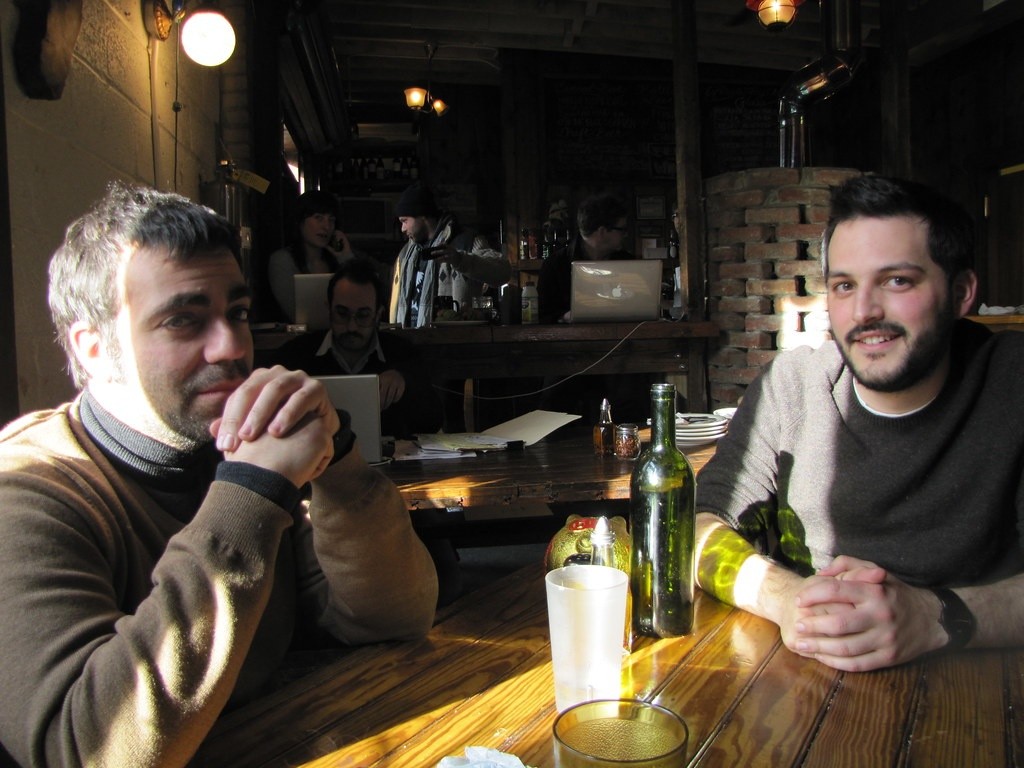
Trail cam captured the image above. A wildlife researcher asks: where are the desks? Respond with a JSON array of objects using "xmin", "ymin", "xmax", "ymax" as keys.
[
  {"xmin": 380, "ymin": 443, "xmax": 718, "ymax": 561},
  {"xmin": 200, "ymin": 552, "xmax": 1024, "ymax": 768}
]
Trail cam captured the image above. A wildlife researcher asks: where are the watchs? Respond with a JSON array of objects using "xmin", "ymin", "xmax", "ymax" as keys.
[{"xmin": 928, "ymin": 582, "xmax": 976, "ymax": 645}]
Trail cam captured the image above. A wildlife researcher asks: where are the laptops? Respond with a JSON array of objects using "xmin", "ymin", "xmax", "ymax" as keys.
[
  {"xmin": 560, "ymin": 257, "xmax": 664, "ymax": 324},
  {"xmin": 307, "ymin": 374, "xmax": 383, "ymax": 463},
  {"xmin": 294, "ymin": 271, "xmax": 338, "ymax": 332}
]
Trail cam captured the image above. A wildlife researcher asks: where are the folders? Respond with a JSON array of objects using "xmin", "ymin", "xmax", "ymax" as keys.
[{"xmin": 412, "ymin": 410, "xmax": 582, "ymax": 451}]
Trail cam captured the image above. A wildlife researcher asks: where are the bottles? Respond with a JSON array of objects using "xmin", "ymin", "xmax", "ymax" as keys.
[
  {"xmin": 614, "ymin": 423, "xmax": 642, "ymax": 460},
  {"xmin": 629, "ymin": 383, "xmax": 697, "ymax": 639},
  {"xmin": 520, "ymin": 274, "xmax": 539, "ymax": 325},
  {"xmin": 519, "ymin": 226, "xmax": 572, "ymax": 261},
  {"xmin": 669, "ymin": 228, "xmax": 678, "ymax": 258},
  {"xmin": 593, "ymin": 398, "xmax": 618, "ymax": 458}
]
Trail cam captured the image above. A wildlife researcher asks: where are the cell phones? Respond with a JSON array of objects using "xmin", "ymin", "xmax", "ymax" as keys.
[{"xmin": 422, "ymin": 246, "xmax": 445, "ymax": 261}]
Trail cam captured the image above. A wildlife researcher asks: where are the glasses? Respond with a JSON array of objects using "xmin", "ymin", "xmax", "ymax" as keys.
[
  {"xmin": 604, "ymin": 222, "xmax": 629, "ymax": 236},
  {"xmin": 330, "ymin": 303, "xmax": 378, "ymax": 329}
]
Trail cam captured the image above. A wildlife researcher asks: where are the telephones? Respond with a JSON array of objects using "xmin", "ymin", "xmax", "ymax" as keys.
[{"xmin": 328, "ymin": 238, "xmax": 343, "ymax": 251}]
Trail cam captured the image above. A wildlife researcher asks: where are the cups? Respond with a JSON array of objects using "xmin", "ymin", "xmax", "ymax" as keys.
[
  {"xmin": 552, "ymin": 699, "xmax": 688, "ymax": 768},
  {"xmin": 473, "ymin": 296, "xmax": 497, "ymax": 319},
  {"xmin": 545, "ymin": 564, "xmax": 629, "ymax": 715},
  {"xmin": 431, "ymin": 295, "xmax": 460, "ymax": 323}
]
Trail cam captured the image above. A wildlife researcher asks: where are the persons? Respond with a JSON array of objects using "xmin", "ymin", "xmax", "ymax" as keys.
[
  {"xmin": 272, "ymin": 186, "xmax": 681, "ymax": 444},
  {"xmin": 688, "ymin": 175, "xmax": 1024, "ymax": 670},
  {"xmin": 0, "ymin": 183, "xmax": 439, "ymax": 768}
]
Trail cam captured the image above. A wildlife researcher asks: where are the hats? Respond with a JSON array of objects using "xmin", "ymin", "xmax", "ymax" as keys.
[{"xmin": 394, "ymin": 184, "xmax": 442, "ymax": 218}]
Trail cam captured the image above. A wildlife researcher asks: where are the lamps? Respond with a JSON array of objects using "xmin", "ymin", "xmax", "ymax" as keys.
[
  {"xmin": 142, "ymin": 0, "xmax": 236, "ymax": 67},
  {"xmin": 746, "ymin": 0, "xmax": 806, "ymax": 32},
  {"xmin": 403, "ymin": 40, "xmax": 449, "ymax": 117}
]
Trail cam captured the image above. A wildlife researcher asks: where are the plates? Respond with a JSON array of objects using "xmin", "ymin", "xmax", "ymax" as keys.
[
  {"xmin": 434, "ymin": 320, "xmax": 489, "ymax": 325},
  {"xmin": 645, "ymin": 407, "xmax": 738, "ymax": 446}
]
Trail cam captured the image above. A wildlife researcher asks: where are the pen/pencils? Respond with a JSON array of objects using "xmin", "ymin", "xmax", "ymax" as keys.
[{"xmin": 404, "ymin": 437, "xmax": 419, "ymax": 440}]
